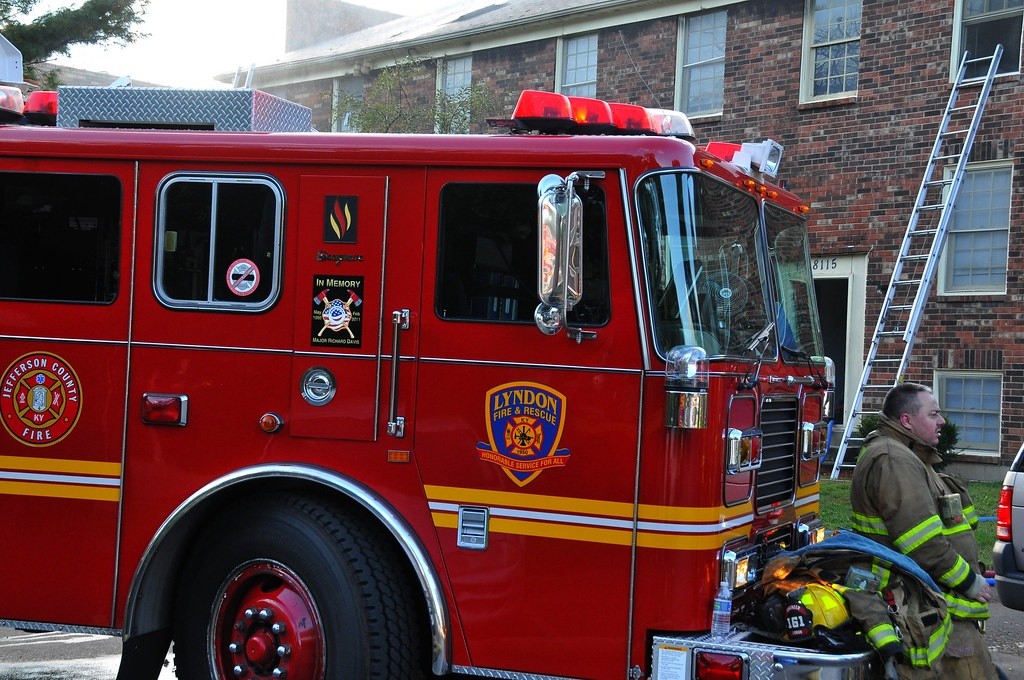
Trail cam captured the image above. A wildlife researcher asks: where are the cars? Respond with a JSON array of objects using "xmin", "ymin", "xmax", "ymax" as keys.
[{"xmin": 991, "ymin": 439, "xmax": 1024, "ymax": 615}]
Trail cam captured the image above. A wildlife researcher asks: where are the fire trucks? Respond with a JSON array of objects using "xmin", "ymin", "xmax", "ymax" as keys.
[{"xmin": 0, "ymin": 35, "xmax": 891, "ymax": 680}]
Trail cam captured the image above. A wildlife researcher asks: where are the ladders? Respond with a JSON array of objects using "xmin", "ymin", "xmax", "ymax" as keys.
[{"xmin": 829, "ymin": 44, "xmax": 1006, "ymax": 485}]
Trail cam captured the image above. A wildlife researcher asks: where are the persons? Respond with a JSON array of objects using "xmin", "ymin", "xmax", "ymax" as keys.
[{"xmin": 851, "ymin": 383, "xmax": 1009, "ymax": 680}]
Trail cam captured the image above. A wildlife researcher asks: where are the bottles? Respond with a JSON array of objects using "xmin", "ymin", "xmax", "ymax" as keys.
[{"xmin": 711, "ymin": 581, "xmax": 732, "ymax": 640}]
